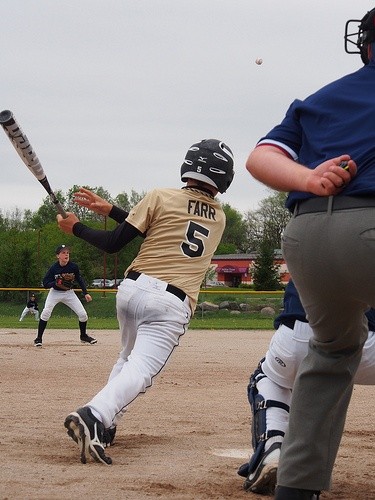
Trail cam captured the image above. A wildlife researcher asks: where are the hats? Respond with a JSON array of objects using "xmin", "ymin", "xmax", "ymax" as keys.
[
  {"xmin": 55, "ymin": 243, "xmax": 72, "ymax": 254},
  {"xmin": 31, "ymin": 293, "xmax": 35, "ymax": 297}
]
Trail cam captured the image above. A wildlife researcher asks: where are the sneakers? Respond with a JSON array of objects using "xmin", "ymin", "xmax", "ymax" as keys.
[
  {"xmin": 80, "ymin": 334, "xmax": 97, "ymax": 344},
  {"xmin": 242, "ymin": 461, "xmax": 278, "ymax": 495},
  {"xmin": 33, "ymin": 338, "xmax": 43, "ymax": 346},
  {"xmin": 63, "ymin": 407, "xmax": 116, "ymax": 467}
]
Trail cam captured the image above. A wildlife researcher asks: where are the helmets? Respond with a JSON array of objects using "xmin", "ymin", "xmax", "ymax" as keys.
[
  {"xmin": 181, "ymin": 139, "xmax": 235, "ymax": 194},
  {"xmin": 359, "ymin": 7, "xmax": 375, "ymax": 45}
]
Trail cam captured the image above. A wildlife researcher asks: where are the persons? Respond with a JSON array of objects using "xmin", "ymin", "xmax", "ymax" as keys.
[
  {"xmin": 56, "ymin": 139, "xmax": 236, "ymax": 465},
  {"xmin": 18, "ymin": 293, "xmax": 40, "ymax": 322},
  {"xmin": 237, "ymin": 277, "xmax": 375, "ymax": 492},
  {"xmin": 245, "ymin": 8, "xmax": 375, "ymax": 500},
  {"xmin": 34, "ymin": 244, "xmax": 97, "ymax": 346}
]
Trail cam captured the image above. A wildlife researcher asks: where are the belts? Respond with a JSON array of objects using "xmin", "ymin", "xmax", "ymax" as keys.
[
  {"xmin": 281, "ymin": 319, "xmax": 296, "ymax": 330},
  {"xmin": 125, "ymin": 270, "xmax": 186, "ymax": 302},
  {"xmin": 294, "ymin": 193, "xmax": 375, "ymax": 215}
]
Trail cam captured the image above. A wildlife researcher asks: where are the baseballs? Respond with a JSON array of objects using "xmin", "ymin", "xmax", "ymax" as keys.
[{"xmin": 255, "ymin": 58, "xmax": 263, "ymax": 65}]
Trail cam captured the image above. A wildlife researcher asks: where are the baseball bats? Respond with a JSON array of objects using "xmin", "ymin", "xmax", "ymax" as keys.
[{"xmin": 0, "ymin": 110, "xmax": 66, "ymax": 220}]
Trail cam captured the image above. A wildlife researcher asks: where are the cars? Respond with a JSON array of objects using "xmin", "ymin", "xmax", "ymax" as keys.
[
  {"xmin": 90, "ymin": 278, "xmax": 111, "ymax": 289},
  {"xmin": 109, "ymin": 279, "xmax": 124, "ymax": 289}
]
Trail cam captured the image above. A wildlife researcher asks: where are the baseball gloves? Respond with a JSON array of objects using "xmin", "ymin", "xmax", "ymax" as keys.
[{"xmin": 54, "ymin": 273, "xmax": 74, "ymax": 291}]
